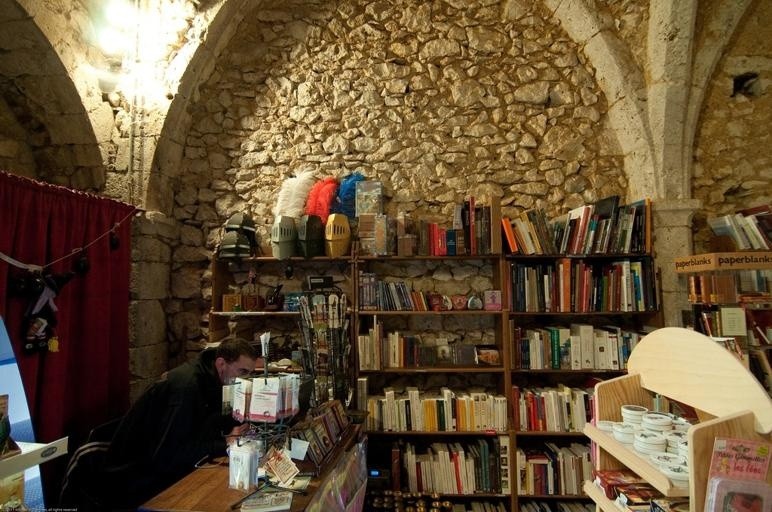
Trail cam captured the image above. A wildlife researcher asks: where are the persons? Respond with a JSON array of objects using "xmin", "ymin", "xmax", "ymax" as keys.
[{"xmin": 108, "ymin": 337, "xmax": 259, "ymax": 506}]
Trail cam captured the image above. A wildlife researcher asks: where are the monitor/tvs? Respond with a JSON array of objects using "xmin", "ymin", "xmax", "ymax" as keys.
[{"xmin": 275, "ymin": 377, "xmax": 315, "ymax": 431}]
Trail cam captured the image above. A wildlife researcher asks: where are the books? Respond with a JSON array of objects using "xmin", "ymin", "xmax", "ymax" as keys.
[
  {"xmin": 501, "ymin": 194, "xmax": 645, "ymax": 255},
  {"xmin": 509, "ymin": 319, "xmax": 658, "ymax": 371},
  {"xmin": 354, "ymin": 193, "xmax": 491, "ymax": 256},
  {"xmin": 685, "ymin": 211, "xmax": 772, "ymax": 399},
  {"xmin": 390, "ymin": 435, "xmax": 510, "ymax": 495},
  {"xmin": 519, "ymin": 501, "xmax": 597, "ymax": 511},
  {"xmin": 288, "ymin": 399, "xmax": 351, "ymax": 467},
  {"xmin": 511, "ymin": 376, "xmax": 605, "ymax": 432},
  {"xmin": 452, "ymin": 501, "xmax": 506, "ymax": 512},
  {"xmin": 357, "ymin": 374, "xmax": 507, "ymax": 432},
  {"xmin": 510, "ymin": 257, "xmax": 657, "ymax": 313},
  {"xmin": 357, "ymin": 261, "xmax": 429, "ymax": 311},
  {"xmin": 517, "ymin": 439, "xmax": 596, "ymax": 495},
  {"xmin": 357, "ymin": 314, "xmax": 421, "ymax": 370}
]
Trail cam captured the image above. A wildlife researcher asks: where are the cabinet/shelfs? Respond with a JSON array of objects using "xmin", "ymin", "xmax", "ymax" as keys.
[
  {"xmin": 514, "ymin": 252, "xmax": 656, "ymax": 512},
  {"xmin": 678, "ymin": 251, "xmax": 772, "ymax": 395},
  {"xmin": 582, "ymin": 325, "xmax": 772, "ymax": 511},
  {"xmin": 203, "ymin": 246, "xmax": 355, "ymax": 376},
  {"xmin": 357, "ymin": 241, "xmax": 514, "ymax": 511},
  {"xmin": 294, "ymin": 274, "xmax": 353, "ymax": 435}
]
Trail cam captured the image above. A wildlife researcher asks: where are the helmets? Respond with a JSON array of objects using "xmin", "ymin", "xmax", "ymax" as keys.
[
  {"xmin": 325, "ymin": 213, "xmax": 352, "ymax": 260},
  {"xmin": 219, "ymin": 213, "xmax": 255, "ymax": 257},
  {"xmin": 298, "ymin": 214, "xmax": 324, "ymax": 258},
  {"xmin": 271, "ymin": 215, "xmax": 296, "ymax": 260}
]
